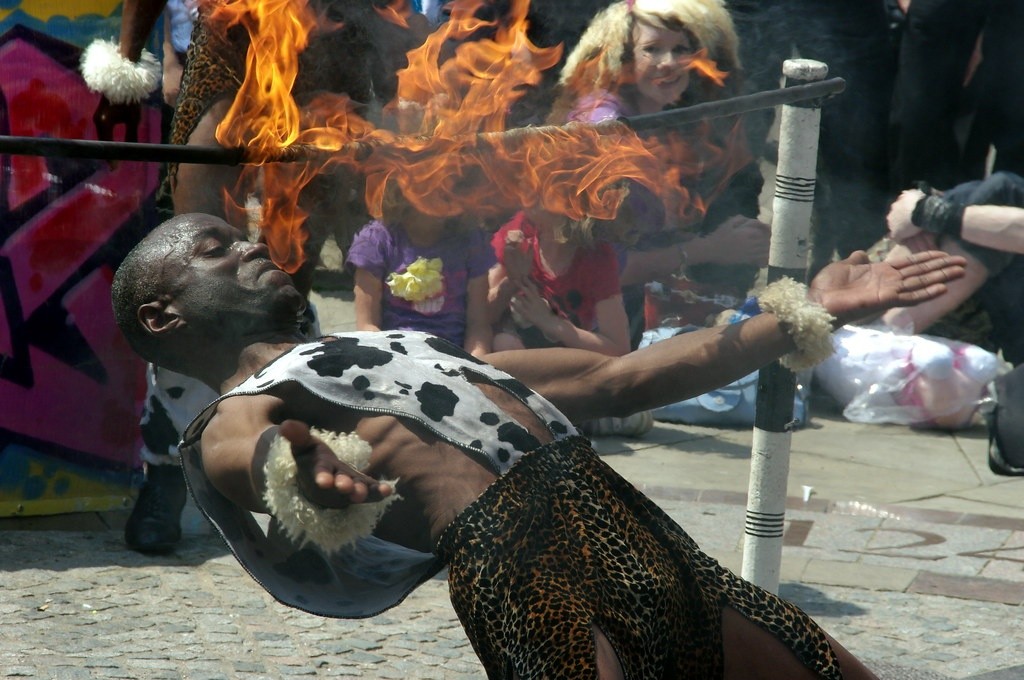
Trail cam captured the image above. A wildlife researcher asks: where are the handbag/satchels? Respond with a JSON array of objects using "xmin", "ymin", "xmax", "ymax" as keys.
[
  {"xmin": 970, "ymin": 363, "xmax": 1024, "ymax": 476},
  {"xmin": 816, "ymin": 323, "xmax": 1013, "ymax": 428},
  {"xmin": 637, "ymin": 322, "xmax": 809, "ymax": 429}
]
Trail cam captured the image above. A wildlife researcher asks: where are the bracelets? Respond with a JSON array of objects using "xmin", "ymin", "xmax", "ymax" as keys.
[
  {"xmin": 909, "ymin": 192, "xmax": 964, "ymax": 241},
  {"xmin": 717, "ymin": 273, "xmax": 841, "ymax": 373},
  {"xmin": 80, "ymin": 34, "xmax": 162, "ymax": 104},
  {"xmin": 264, "ymin": 426, "xmax": 403, "ymax": 557}
]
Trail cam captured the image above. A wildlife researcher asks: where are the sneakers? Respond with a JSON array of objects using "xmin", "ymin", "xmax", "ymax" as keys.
[{"xmin": 581, "ymin": 409, "xmax": 653, "ymax": 437}]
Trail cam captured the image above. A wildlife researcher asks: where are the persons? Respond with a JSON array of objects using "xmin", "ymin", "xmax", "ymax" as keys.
[
  {"xmin": 92, "ymin": 0, "xmax": 1024, "ymax": 556},
  {"xmin": 110, "ymin": 212, "xmax": 966, "ymax": 680}
]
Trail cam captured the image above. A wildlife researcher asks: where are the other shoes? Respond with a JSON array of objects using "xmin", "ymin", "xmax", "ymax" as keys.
[{"xmin": 124, "ymin": 475, "xmax": 188, "ymax": 556}]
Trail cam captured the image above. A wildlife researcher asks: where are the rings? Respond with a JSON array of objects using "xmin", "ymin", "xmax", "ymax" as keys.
[{"xmin": 102, "ymin": 112, "xmax": 107, "ymax": 120}]
